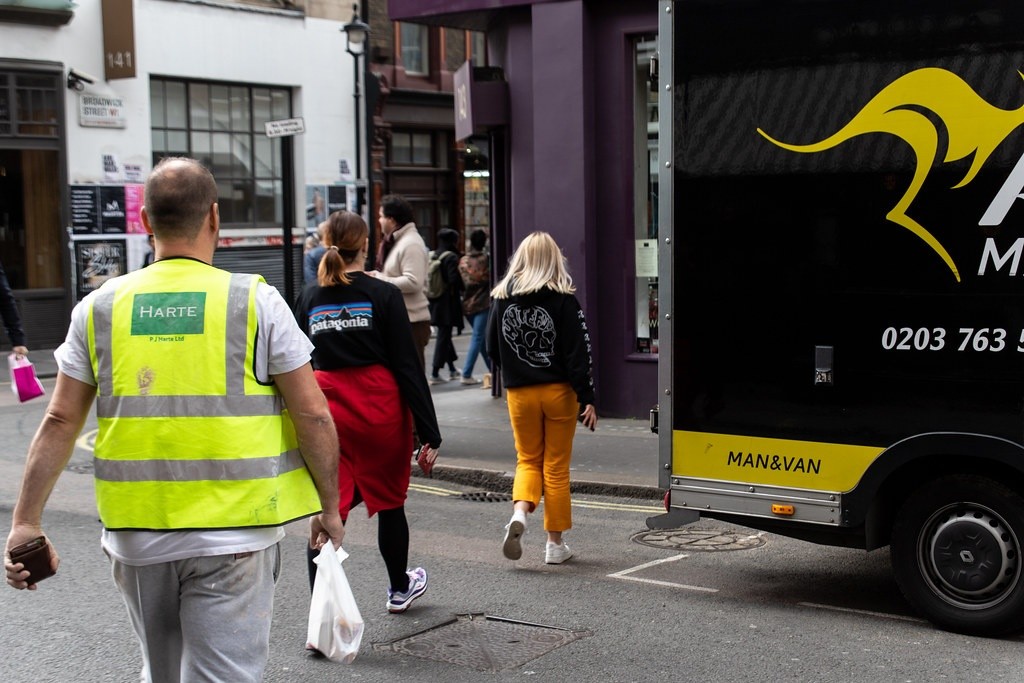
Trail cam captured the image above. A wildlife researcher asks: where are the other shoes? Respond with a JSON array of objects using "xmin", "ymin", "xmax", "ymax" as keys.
[
  {"xmin": 429, "ymin": 374, "xmax": 449, "ymax": 384},
  {"xmin": 459, "ymin": 376, "xmax": 483, "ymax": 385},
  {"xmin": 448, "ymin": 371, "xmax": 462, "ymax": 380}
]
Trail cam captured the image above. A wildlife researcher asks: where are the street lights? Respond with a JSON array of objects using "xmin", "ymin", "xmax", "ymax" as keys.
[{"xmin": 338, "ymin": 1, "xmax": 373, "ymax": 219}]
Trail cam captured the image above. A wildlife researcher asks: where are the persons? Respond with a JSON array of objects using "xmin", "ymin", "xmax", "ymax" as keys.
[
  {"xmin": 487, "ymin": 230, "xmax": 597, "ymax": 563},
  {"xmin": 0, "ymin": 265, "xmax": 28, "ymax": 360},
  {"xmin": 5, "ymin": 156, "xmax": 343, "ymax": 683},
  {"xmin": 302, "ymin": 200, "xmax": 495, "ymax": 385},
  {"xmin": 281, "ymin": 211, "xmax": 442, "ymax": 660}
]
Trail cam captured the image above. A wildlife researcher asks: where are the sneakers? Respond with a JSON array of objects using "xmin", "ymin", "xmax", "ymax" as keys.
[
  {"xmin": 502, "ymin": 510, "xmax": 528, "ymax": 561},
  {"xmin": 385, "ymin": 567, "xmax": 429, "ymax": 614},
  {"xmin": 545, "ymin": 540, "xmax": 573, "ymax": 564}
]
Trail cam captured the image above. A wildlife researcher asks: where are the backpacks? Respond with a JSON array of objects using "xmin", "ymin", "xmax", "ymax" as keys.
[{"xmin": 422, "ymin": 251, "xmax": 455, "ymax": 300}]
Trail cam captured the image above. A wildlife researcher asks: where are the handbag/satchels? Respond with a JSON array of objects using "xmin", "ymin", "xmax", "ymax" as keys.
[
  {"xmin": 6, "ymin": 353, "xmax": 47, "ymax": 404},
  {"xmin": 304, "ymin": 537, "xmax": 366, "ymax": 665}
]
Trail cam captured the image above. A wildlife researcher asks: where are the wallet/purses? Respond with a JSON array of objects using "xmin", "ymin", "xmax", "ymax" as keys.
[{"xmin": 8, "ymin": 535, "xmax": 57, "ymax": 588}]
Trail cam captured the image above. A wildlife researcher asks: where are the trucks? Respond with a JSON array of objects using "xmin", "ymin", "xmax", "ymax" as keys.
[{"xmin": 651, "ymin": 1, "xmax": 1024, "ymax": 635}]
